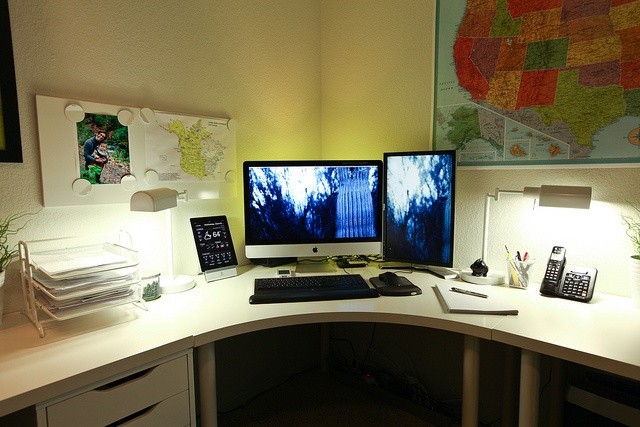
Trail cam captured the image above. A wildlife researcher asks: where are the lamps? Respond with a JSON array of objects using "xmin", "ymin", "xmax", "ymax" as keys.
[
  {"xmin": 460, "ymin": 182, "xmax": 592, "ymax": 285},
  {"xmin": 130, "ymin": 186, "xmax": 197, "ymax": 292}
]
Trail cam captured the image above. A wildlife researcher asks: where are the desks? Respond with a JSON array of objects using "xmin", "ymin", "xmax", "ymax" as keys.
[{"xmin": 0, "ymin": 262, "xmax": 640, "ymax": 425}]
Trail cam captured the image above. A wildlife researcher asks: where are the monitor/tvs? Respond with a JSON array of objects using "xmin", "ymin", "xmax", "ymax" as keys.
[
  {"xmin": 242, "ymin": 161, "xmax": 383, "ymax": 274},
  {"xmin": 378, "ymin": 150, "xmax": 459, "ymax": 280}
]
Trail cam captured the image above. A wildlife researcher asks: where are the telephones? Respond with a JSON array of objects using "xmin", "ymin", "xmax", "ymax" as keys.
[{"xmin": 540, "ymin": 246, "xmax": 598, "ymax": 302}]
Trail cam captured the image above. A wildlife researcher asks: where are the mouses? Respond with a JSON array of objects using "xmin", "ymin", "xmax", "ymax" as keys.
[{"xmin": 379, "ymin": 272, "xmax": 401, "ymax": 286}]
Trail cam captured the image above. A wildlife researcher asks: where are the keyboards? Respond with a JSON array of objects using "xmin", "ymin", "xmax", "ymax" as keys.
[{"xmin": 249, "ymin": 274, "xmax": 379, "ymax": 305}]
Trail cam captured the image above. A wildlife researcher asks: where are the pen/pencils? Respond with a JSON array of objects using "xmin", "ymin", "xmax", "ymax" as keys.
[
  {"xmin": 523, "ymin": 251, "xmax": 528, "ymax": 261},
  {"xmin": 506, "ymin": 256, "xmax": 525, "ymax": 287},
  {"xmin": 451, "ymin": 287, "xmax": 487, "ymax": 299},
  {"xmin": 515, "ymin": 250, "xmax": 525, "ymax": 278},
  {"xmin": 505, "ymin": 245, "xmax": 527, "ymax": 289}
]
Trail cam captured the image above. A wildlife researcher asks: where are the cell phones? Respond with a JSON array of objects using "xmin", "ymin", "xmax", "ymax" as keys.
[{"xmin": 276, "ymin": 268, "xmax": 291, "ymax": 278}]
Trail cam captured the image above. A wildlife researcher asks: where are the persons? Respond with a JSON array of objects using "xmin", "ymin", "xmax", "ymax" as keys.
[
  {"xmin": 89, "ymin": 142, "xmax": 109, "ymax": 168},
  {"xmin": 83, "ymin": 131, "xmax": 108, "ymax": 183}
]
